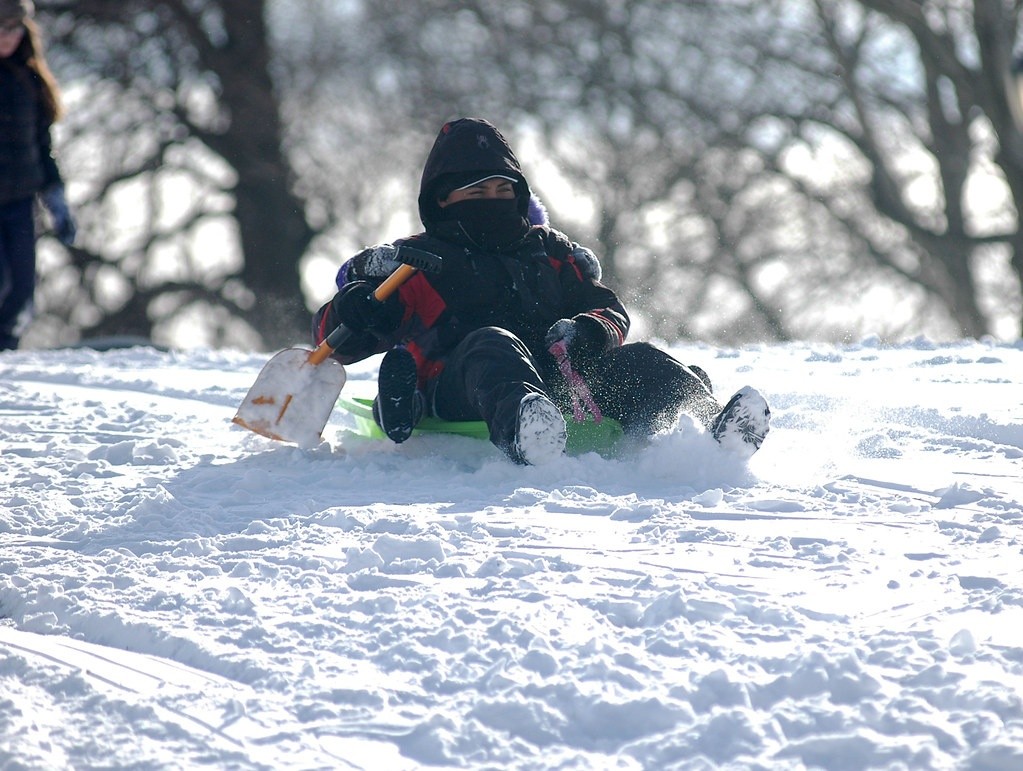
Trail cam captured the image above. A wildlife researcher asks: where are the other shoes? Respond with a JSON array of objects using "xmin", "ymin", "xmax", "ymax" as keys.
[
  {"xmin": 712, "ymin": 385, "xmax": 771, "ymax": 466},
  {"xmin": 515, "ymin": 392, "xmax": 566, "ymax": 466}
]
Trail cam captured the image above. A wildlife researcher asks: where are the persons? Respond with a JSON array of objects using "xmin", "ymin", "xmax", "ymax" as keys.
[{"xmin": 314, "ymin": 118, "xmax": 776, "ymax": 470}]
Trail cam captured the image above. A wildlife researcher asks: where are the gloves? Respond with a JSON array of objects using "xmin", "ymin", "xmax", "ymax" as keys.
[
  {"xmin": 336, "ymin": 286, "xmax": 387, "ymax": 335},
  {"xmin": 57, "ymin": 217, "xmax": 77, "ymax": 245},
  {"xmin": 544, "ymin": 317, "xmax": 594, "ymax": 357}
]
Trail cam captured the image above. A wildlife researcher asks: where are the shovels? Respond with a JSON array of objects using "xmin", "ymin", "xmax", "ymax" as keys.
[{"xmin": 231, "ymin": 263, "xmax": 416, "ymax": 443}]
